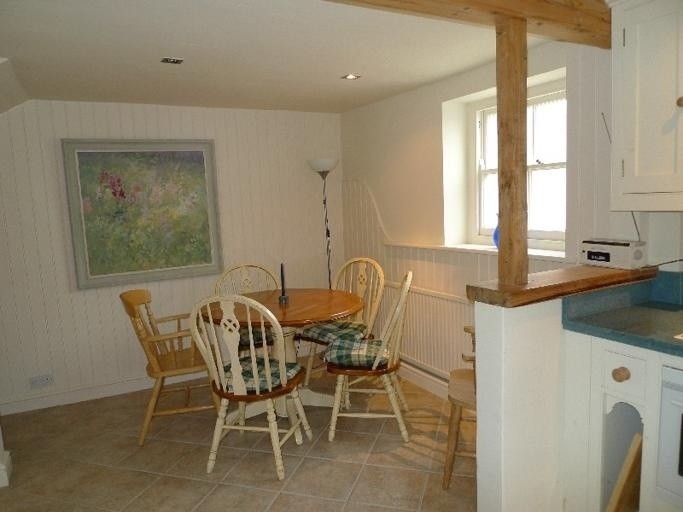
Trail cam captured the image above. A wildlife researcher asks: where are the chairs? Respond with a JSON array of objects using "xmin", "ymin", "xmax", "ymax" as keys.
[{"xmin": 440, "ymin": 323, "xmax": 475, "ymax": 492}]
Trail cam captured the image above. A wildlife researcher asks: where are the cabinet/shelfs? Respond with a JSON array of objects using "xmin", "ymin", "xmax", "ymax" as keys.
[
  {"xmin": 584, "ymin": 334, "xmax": 662, "ymax": 511},
  {"xmin": 601, "ymin": 0, "xmax": 683, "ymax": 216}
]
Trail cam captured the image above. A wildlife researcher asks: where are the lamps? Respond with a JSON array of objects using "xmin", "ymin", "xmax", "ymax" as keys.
[{"xmin": 304, "ymin": 156, "xmax": 342, "ymax": 289}]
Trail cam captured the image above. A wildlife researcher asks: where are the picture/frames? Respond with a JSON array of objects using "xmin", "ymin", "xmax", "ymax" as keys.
[{"xmin": 58, "ymin": 134, "xmax": 227, "ymax": 292}]
